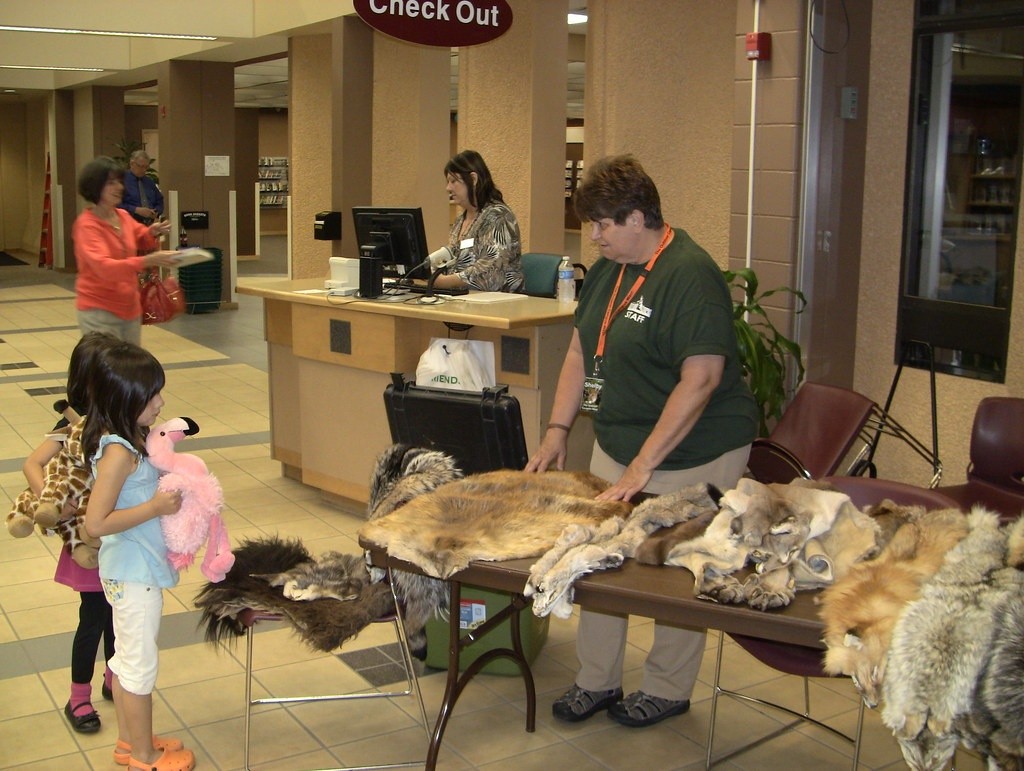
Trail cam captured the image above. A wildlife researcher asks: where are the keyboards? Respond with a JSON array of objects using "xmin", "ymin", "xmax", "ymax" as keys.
[{"xmin": 384, "ymin": 281, "xmax": 470, "ymax": 296}]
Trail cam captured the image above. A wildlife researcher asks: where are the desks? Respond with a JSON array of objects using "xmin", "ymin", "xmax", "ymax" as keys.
[{"xmin": 359, "ymin": 472, "xmax": 848, "ymax": 771}]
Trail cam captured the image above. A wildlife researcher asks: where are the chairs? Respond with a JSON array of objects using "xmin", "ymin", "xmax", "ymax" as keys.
[
  {"xmin": 236, "ymin": 447, "xmax": 431, "ymax": 771},
  {"xmin": 705, "ymin": 382, "xmax": 1024, "ymax": 771},
  {"xmin": 520, "ymin": 254, "xmax": 561, "ymax": 296}
]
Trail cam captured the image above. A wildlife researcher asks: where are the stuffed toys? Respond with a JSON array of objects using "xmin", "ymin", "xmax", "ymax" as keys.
[
  {"xmin": 146, "ymin": 417, "xmax": 235, "ymax": 582},
  {"xmin": 5, "ymin": 398, "xmax": 102, "ymax": 569}
]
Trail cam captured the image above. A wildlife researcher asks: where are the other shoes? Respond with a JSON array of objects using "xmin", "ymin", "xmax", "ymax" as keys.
[
  {"xmin": 102, "ymin": 681, "xmax": 113, "ymax": 699},
  {"xmin": 64, "ymin": 699, "xmax": 101, "ymax": 732}
]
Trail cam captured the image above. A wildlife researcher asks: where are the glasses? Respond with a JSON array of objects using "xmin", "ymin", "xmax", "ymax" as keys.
[{"xmin": 134, "ymin": 162, "xmax": 150, "ymax": 171}]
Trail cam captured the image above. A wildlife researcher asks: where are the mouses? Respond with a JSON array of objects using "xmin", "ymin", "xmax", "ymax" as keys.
[{"xmin": 402, "ymin": 279, "xmax": 415, "ymax": 284}]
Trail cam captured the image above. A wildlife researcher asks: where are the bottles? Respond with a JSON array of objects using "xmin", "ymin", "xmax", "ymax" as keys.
[
  {"xmin": 180, "ymin": 225, "xmax": 187, "ymax": 247},
  {"xmin": 558, "ymin": 256, "xmax": 576, "ymax": 302}
]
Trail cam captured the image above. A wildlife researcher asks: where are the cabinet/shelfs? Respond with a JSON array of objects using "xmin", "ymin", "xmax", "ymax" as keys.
[
  {"xmin": 964, "ymin": 150, "xmax": 1017, "ymax": 240},
  {"xmin": 259, "ymin": 157, "xmax": 287, "ymax": 208}
]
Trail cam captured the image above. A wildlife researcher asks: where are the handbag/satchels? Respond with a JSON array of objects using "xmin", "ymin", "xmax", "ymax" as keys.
[{"xmin": 135, "ymin": 237, "xmax": 187, "ymax": 324}]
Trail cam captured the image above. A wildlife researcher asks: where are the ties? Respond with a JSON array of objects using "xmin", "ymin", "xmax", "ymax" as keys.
[{"xmin": 137, "ymin": 179, "xmax": 151, "ymax": 225}]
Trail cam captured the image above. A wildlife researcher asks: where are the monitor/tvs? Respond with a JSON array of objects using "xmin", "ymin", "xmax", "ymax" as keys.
[{"xmin": 352, "ymin": 206, "xmax": 434, "ymax": 298}]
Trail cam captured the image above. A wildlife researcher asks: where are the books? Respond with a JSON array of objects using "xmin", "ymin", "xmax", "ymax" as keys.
[
  {"xmin": 259, "ymin": 157, "xmax": 288, "ymax": 204},
  {"xmin": 170, "ymin": 247, "xmax": 213, "ymax": 267}
]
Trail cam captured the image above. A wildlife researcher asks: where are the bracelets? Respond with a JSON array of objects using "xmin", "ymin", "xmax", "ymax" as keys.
[{"xmin": 547, "ymin": 424, "xmax": 570, "ymax": 432}]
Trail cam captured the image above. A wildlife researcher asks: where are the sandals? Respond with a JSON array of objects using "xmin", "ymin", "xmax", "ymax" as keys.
[
  {"xmin": 124, "ymin": 747, "xmax": 195, "ymax": 771},
  {"xmin": 113, "ymin": 735, "xmax": 184, "ymax": 765},
  {"xmin": 606, "ymin": 687, "xmax": 692, "ymax": 727},
  {"xmin": 553, "ymin": 682, "xmax": 623, "ymax": 723}
]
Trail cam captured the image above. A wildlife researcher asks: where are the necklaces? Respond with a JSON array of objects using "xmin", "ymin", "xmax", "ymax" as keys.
[{"xmin": 115, "ymin": 226, "xmax": 120, "ymax": 230}]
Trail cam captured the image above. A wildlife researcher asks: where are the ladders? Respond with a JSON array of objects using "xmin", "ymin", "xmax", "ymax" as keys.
[{"xmin": 38, "ymin": 152, "xmax": 53, "ymax": 270}]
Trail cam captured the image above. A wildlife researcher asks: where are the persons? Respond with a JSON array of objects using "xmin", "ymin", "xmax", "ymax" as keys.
[
  {"xmin": 80, "ymin": 343, "xmax": 195, "ymax": 771},
  {"xmin": 71, "ymin": 156, "xmax": 181, "ymax": 346},
  {"xmin": 22, "ymin": 331, "xmax": 119, "ymax": 734},
  {"xmin": 116, "ymin": 150, "xmax": 164, "ymax": 284},
  {"xmin": 524, "ymin": 155, "xmax": 760, "ymax": 726},
  {"xmin": 430, "ymin": 150, "xmax": 525, "ymax": 292}
]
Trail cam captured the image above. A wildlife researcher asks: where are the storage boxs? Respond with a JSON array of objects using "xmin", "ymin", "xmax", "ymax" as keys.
[{"xmin": 179, "ymin": 247, "xmax": 223, "ymax": 313}]
